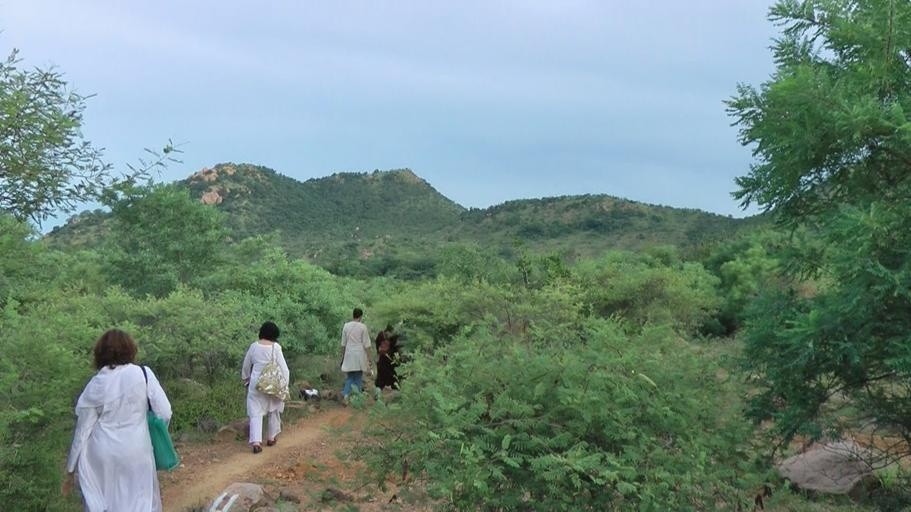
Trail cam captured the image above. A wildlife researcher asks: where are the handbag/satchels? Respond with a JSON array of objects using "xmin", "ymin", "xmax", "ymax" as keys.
[
  {"xmin": 255, "ymin": 360, "xmax": 290, "ymax": 402},
  {"xmin": 146, "ymin": 411, "xmax": 180, "ymax": 471},
  {"xmin": 379, "ymin": 340, "xmax": 390, "ymax": 352}
]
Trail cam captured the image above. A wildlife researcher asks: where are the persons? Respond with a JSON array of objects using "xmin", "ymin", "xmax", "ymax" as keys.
[
  {"xmin": 241, "ymin": 322, "xmax": 290, "ymax": 452},
  {"xmin": 340, "ymin": 308, "xmax": 372, "ymax": 408},
  {"xmin": 375, "ymin": 323, "xmax": 400, "ymax": 399},
  {"xmin": 58, "ymin": 328, "xmax": 174, "ymax": 512}
]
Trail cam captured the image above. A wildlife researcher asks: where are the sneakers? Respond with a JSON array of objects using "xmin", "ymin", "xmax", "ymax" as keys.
[
  {"xmin": 254, "ymin": 445, "xmax": 262, "ymax": 453},
  {"xmin": 268, "ymin": 438, "xmax": 277, "ymax": 446}
]
